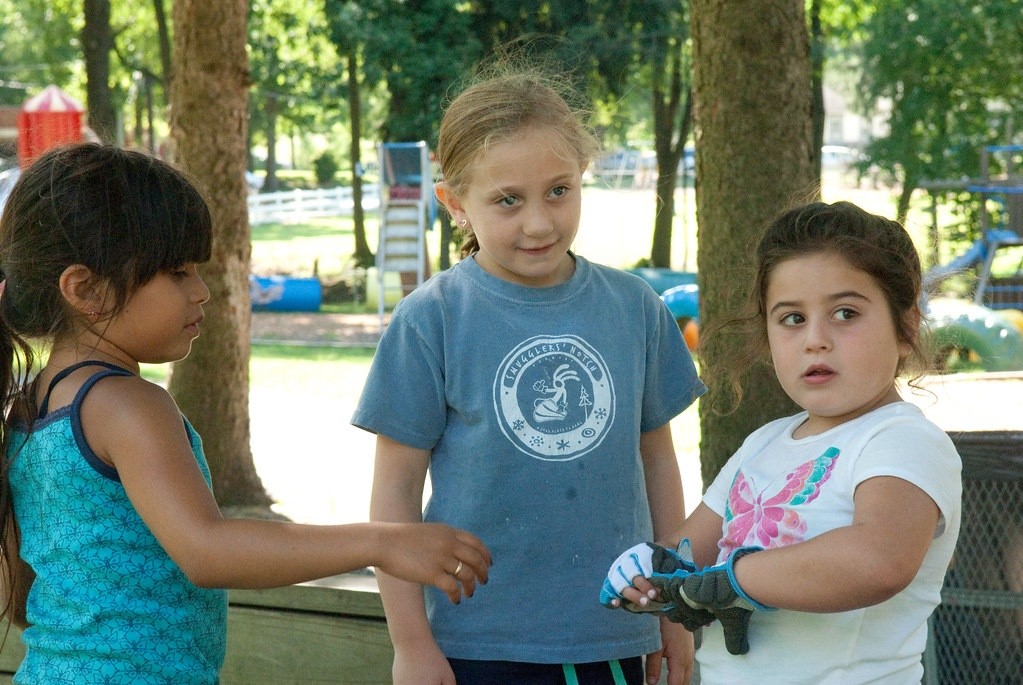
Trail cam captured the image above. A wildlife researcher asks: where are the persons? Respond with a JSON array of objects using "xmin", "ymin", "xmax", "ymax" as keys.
[
  {"xmin": 0, "ymin": 142, "xmax": 492, "ymax": 685},
  {"xmin": 599, "ymin": 201, "xmax": 962, "ymax": 685},
  {"xmin": 349, "ymin": 78, "xmax": 709, "ymax": 685}
]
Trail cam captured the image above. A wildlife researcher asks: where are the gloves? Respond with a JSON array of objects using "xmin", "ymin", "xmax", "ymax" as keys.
[{"xmin": 649, "ymin": 546, "xmax": 778, "ymax": 656}]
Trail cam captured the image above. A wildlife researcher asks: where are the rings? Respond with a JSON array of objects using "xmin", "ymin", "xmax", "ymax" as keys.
[{"xmin": 453, "ymin": 561, "xmax": 463, "ymax": 575}]
